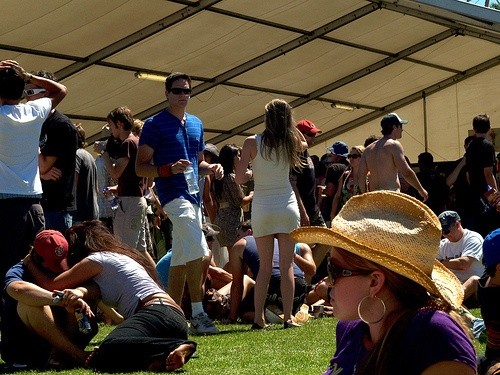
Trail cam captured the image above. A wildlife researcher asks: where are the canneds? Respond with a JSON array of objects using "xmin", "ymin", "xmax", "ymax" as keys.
[{"xmin": 74, "ymin": 308, "xmax": 91, "ymax": 332}]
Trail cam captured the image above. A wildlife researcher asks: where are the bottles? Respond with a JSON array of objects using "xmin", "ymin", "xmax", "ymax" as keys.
[
  {"xmin": 485, "ymin": 184, "xmax": 492, "ymax": 191},
  {"xmin": 183, "ymin": 166, "xmax": 200, "ymax": 195},
  {"xmin": 104, "ymin": 188, "xmax": 119, "ymax": 210}
]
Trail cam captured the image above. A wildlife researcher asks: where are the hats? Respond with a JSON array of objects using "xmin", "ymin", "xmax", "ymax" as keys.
[
  {"xmin": 480, "ymin": 227, "xmax": 500, "ymax": 266},
  {"xmin": 326, "ymin": 141, "xmax": 351, "ymax": 157},
  {"xmin": 288, "ymin": 189, "xmax": 464, "ymax": 310},
  {"xmin": 437, "ymin": 209, "xmax": 462, "ymax": 228},
  {"xmin": 204, "ymin": 142, "xmax": 220, "ymax": 157},
  {"xmin": 380, "ymin": 113, "xmax": 408, "ymax": 125},
  {"xmin": 295, "ymin": 119, "xmax": 322, "ymax": 134},
  {"xmin": 33, "ymin": 229, "xmax": 70, "ymax": 273}
]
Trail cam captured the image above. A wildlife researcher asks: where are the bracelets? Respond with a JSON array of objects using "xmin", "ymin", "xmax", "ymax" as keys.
[{"xmin": 28, "ymin": 74, "xmax": 34, "ymax": 85}]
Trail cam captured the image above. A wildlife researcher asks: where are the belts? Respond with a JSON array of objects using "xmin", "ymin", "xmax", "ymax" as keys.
[{"xmin": 141, "ymin": 296, "xmax": 184, "ymax": 315}]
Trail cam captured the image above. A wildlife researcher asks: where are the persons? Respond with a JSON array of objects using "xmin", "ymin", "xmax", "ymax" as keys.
[
  {"xmin": 0, "ymin": 229, "xmax": 105, "ymax": 370},
  {"xmin": 0, "ymin": 59, "xmax": 69, "ymax": 290},
  {"xmin": 289, "ymin": 189, "xmax": 479, "ymax": 375},
  {"xmin": 92, "ymin": 100, "xmax": 500, "ymax": 317},
  {"xmin": 136, "ymin": 72, "xmax": 226, "ymax": 333},
  {"xmin": 24, "ymin": 220, "xmax": 198, "ymax": 371},
  {"xmin": 23, "ymin": 69, "xmax": 78, "ymax": 237},
  {"xmin": 434, "ymin": 209, "xmax": 487, "ymax": 307},
  {"xmin": 73, "ymin": 120, "xmax": 102, "ymax": 237},
  {"xmin": 478, "ymin": 228, "xmax": 500, "ymax": 360},
  {"xmin": 235, "ymin": 100, "xmax": 304, "ymax": 330}
]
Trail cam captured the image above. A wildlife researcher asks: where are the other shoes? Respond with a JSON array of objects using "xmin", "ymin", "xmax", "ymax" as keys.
[
  {"xmin": 294, "ymin": 303, "xmax": 310, "ymax": 324},
  {"xmin": 283, "ymin": 321, "xmax": 304, "ymax": 329},
  {"xmin": 251, "ymin": 322, "xmax": 270, "ymax": 330},
  {"xmin": 264, "ymin": 304, "xmax": 286, "ymax": 324}
]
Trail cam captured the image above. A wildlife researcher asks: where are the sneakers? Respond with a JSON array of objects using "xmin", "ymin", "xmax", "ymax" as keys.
[
  {"xmin": 189, "ymin": 312, "xmax": 220, "ymax": 336},
  {"xmin": 470, "ymin": 318, "xmax": 487, "ymax": 339}
]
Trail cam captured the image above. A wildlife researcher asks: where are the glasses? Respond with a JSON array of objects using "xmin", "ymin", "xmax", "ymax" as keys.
[
  {"xmin": 441, "ymin": 225, "xmax": 450, "ymax": 234},
  {"xmin": 23, "ymin": 88, "xmax": 46, "ymax": 97},
  {"xmin": 305, "ymin": 132, "xmax": 316, "ymax": 137},
  {"xmin": 326, "ymin": 257, "xmax": 371, "ymax": 286},
  {"xmin": 399, "ymin": 124, "xmax": 403, "ymax": 128},
  {"xmin": 347, "ymin": 153, "xmax": 360, "ymax": 159},
  {"xmin": 170, "ymin": 87, "xmax": 191, "ymax": 95}
]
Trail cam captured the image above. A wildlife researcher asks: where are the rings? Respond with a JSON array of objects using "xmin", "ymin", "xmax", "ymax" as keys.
[{"xmin": 63, "ymin": 295, "xmax": 69, "ymax": 298}]
